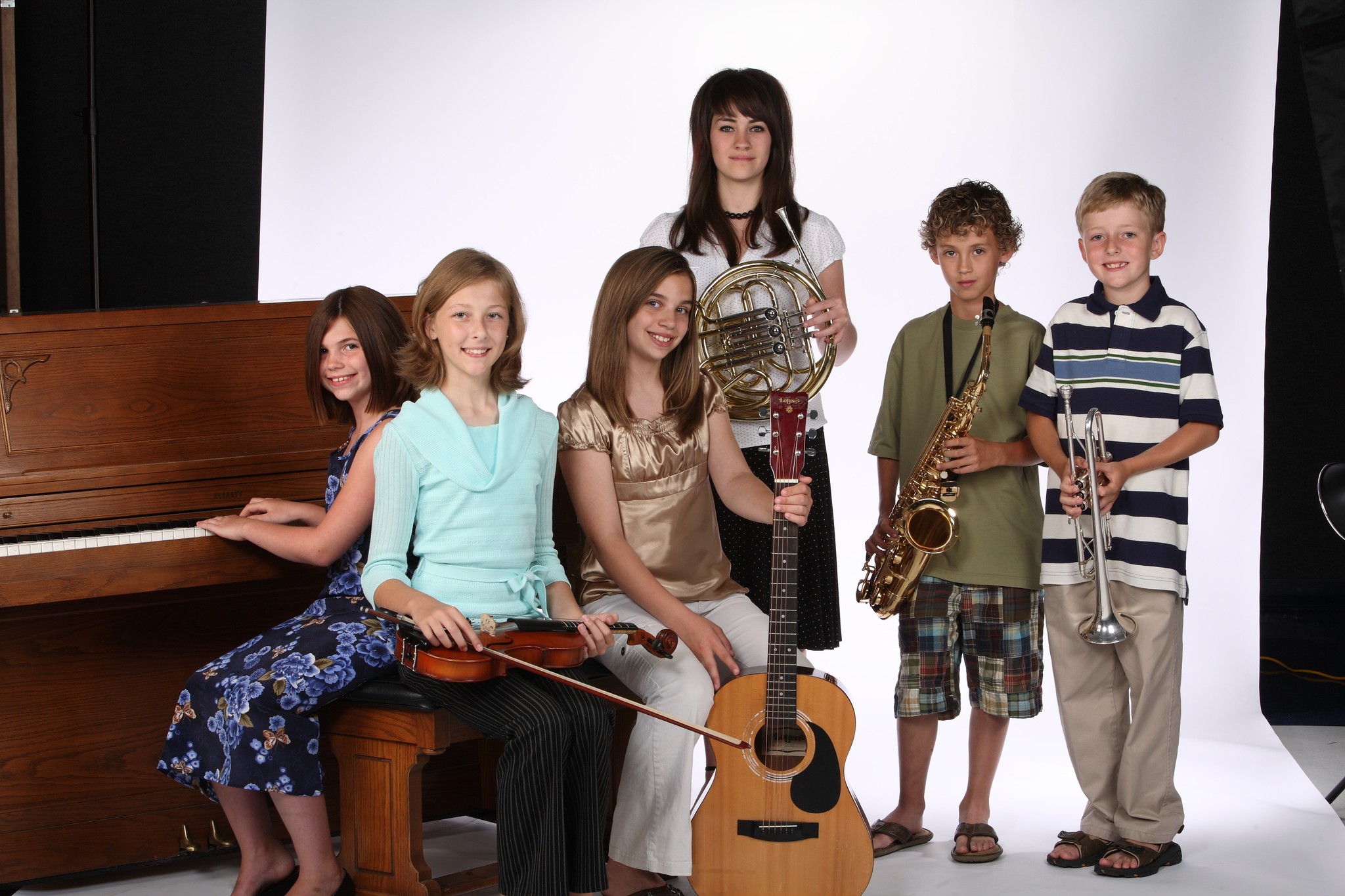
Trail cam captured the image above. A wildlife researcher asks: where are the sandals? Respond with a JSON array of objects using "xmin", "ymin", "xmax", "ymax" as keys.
[
  {"xmin": 1047, "ymin": 829, "xmax": 1121, "ymax": 868},
  {"xmin": 1095, "ymin": 839, "xmax": 1183, "ymax": 879}
]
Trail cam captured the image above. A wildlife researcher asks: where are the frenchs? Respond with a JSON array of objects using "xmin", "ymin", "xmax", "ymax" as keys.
[{"xmin": 696, "ymin": 206, "xmax": 838, "ymax": 421}]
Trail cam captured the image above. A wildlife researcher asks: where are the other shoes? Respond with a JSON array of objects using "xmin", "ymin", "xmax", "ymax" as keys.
[
  {"xmin": 332, "ymin": 868, "xmax": 356, "ymax": 896},
  {"xmin": 256, "ymin": 864, "xmax": 300, "ymax": 896}
]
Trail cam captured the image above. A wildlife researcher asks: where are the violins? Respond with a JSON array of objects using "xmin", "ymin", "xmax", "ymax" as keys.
[{"xmin": 394, "ymin": 613, "xmax": 679, "ymax": 683}]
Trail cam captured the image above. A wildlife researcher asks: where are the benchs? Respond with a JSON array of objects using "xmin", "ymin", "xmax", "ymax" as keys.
[{"xmin": 326, "ymin": 663, "xmax": 642, "ymax": 896}]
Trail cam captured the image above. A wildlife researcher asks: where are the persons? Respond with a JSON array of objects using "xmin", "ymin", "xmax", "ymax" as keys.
[
  {"xmin": 639, "ymin": 68, "xmax": 858, "ymax": 662},
  {"xmin": 359, "ymin": 246, "xmax": 621, "ymax": 896},
  {"xmin": 557, "ymin": 249, "xmax": 814, "ymax": 896},
  {"xmin": 1018, "ymin": 171, "xmax": 1224, "ymax": 878},
  {"xmin": 869, "ymin": 179, "xmax": 1047, "ymax": 864},
  {"xmin": 161, "ymin": 286, "xmax": 415, "ymax": 896}
]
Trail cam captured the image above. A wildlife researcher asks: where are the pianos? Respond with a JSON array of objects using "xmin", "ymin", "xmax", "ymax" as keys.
[{"xmin": 0, "ymin": 294, "xmax": 418, "ymax": 888}]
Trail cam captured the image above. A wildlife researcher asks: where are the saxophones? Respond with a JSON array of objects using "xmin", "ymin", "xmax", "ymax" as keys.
[{"xmin": 856, "ymin": 296, "xmax": 994, "ymax": 620}]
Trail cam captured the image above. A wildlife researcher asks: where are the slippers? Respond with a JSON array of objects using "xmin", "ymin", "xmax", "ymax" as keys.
[
  {"xmin": 950, "ymin": 823, "xmax": 1004, "ymax": 863},
  {"xmin": 869, "ymin": 819, "xmax": 934, "ymax": 858},
  {"xmin": 601, "ymin": 883, "xmax": 684, "ymax": 896}
]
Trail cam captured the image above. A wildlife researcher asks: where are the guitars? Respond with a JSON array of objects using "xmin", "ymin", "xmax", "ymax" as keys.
[{"xmin": 687, "ymin": 391, "xmax": 874, "ymax": 896}]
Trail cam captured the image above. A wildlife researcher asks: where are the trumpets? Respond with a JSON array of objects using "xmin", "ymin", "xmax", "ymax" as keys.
[{"xmin": 1059, "ymin": 385, "xmax": 1138, "ymax": 644}]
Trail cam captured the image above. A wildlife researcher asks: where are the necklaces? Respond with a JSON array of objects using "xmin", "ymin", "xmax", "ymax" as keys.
[{"xmin": 725, "ymin": 211, "xmax": 754, "ymax": 218}]
